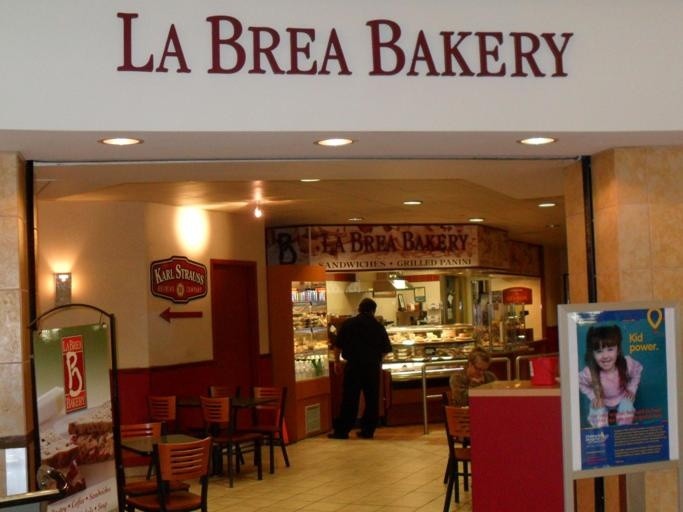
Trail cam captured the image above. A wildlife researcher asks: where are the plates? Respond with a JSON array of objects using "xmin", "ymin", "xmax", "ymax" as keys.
[{"xmin": 411, "ymin": 356, "xmax": 453, "ymax": 361}]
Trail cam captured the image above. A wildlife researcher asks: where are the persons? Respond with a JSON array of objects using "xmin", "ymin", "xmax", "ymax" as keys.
[
  {"xmin": 449, "ymin": 346, "xmax": 498, "ymax": 445},
  {"xmin": 579, "ymin": 325, "xmax": 643, "ymax": 428},
  {"xmin": 327, "ymin": 297, "xmax": 393, "ymax": 439}
]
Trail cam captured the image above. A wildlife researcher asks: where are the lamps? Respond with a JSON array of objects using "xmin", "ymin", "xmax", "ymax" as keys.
[{"xmin": 254, "ymin": 201, "xmax": 262, "ymax": 218}]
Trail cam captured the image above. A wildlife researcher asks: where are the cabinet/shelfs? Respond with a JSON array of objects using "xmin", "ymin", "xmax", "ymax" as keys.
[
  {"xmin": 371, "ymin": 319, "xmax": 496, "ymax": 428},
  {"xmin": 269, "ymin": 267, "xmax": 337, "ymax": 444}
]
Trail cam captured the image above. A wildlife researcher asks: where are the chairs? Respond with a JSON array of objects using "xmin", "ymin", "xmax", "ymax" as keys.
[
  {"xmin": 439, "ymin": 390, "xmax": 471, "ymax": 512},
  {"xmin": 121, "ymin": 379, "xmax": 290, "ymax": 512}
]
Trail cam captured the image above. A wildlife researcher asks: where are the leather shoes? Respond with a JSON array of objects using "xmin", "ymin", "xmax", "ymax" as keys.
[
  {"xmin": 356, "ymin": 431, "xmax": 374, "ymax": 438},
  {"xmin": 327, "ymin": 431, "xmax": 348, "ymax": 439}
]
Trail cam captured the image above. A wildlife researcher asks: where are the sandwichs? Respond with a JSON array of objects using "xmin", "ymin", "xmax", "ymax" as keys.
[
  {"xmin": 39, "ymin": 429, "xmax": 87, "ymax": 505},
  {"xmin": 68, "ymin": 400, "xmax": 114, "ymax": 465}
]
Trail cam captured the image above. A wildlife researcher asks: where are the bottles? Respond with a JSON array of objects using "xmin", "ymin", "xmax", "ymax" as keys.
[
  {"xmin": 295, "ymin": 358, "xmax": 322, "ymax": 379},
  {"xmin": 297, "ymin": 288, "xmax": 318, "ymax": 302}
]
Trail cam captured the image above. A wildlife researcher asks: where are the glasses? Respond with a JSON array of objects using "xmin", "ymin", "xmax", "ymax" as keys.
[{"xmin": 473, "ymin": 363, "xmax": 490, "ymax": 371}]
